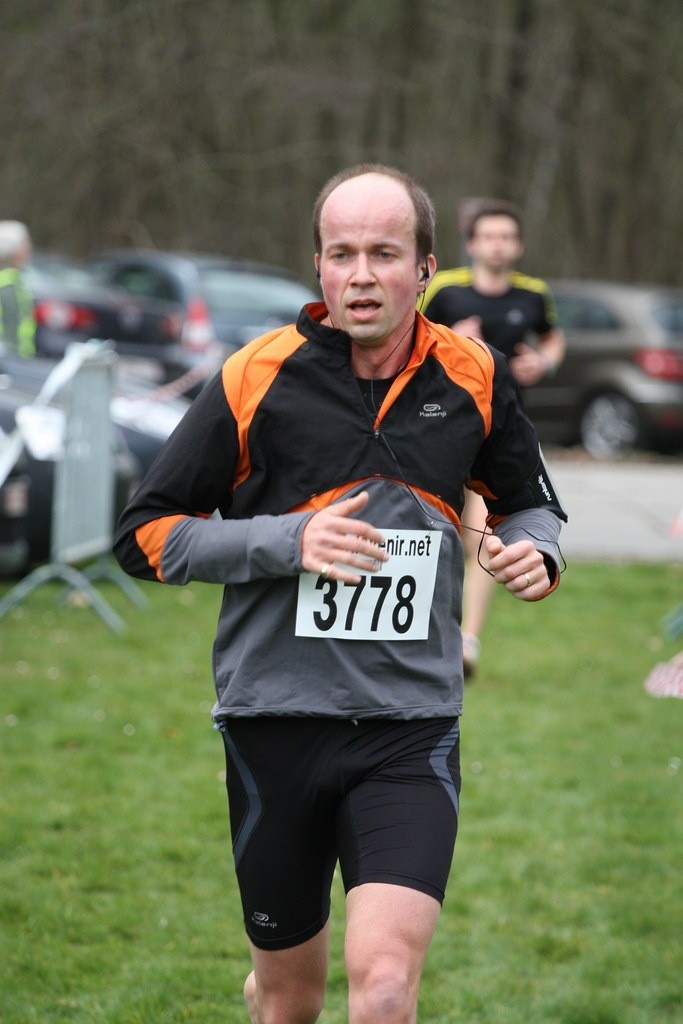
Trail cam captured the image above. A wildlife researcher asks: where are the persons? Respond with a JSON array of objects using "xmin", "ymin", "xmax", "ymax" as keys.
[
  {"xmin": 112, "ymin": 161, "xmax": 569, "ymax": 1023},
  {"xmin": 415, "ymin": 198, "xmax": 564, "ymax": 668},
  {"xmin": 0, "ymin": 219, "xmax": 40, "ymax": 357}
]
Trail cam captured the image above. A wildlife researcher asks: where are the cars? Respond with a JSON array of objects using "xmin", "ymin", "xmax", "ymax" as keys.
[
  {"xmin": 0, "ymin": 242, "xmax": 326, "ymax": 580},
  {"xmin": 520, "ymin": 275, "xmax": 683, "ymax": 463}
]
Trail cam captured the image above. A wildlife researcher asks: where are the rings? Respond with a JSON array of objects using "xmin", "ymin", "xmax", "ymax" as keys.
[
  {"xmin": 321, "ymin": 564, "xmax": 328, "ymax": 577},
  {"xmin": 525, "ymin": 573, "xmax": 531, "ymax": 586}
]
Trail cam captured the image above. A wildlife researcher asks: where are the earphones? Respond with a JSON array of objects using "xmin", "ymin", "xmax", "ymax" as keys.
[
  {"xmin": 419, "ymin": 264, "xmax": 430, "ymax": 283},
  {"xmin": 316, "ymin": 268, "xmax": 321, "ymax": 278}
]
